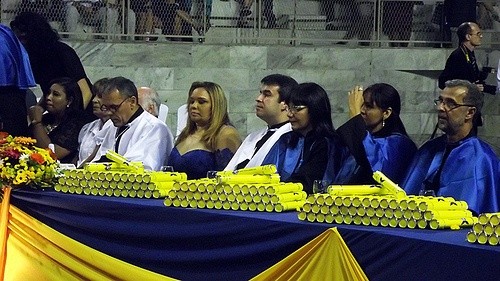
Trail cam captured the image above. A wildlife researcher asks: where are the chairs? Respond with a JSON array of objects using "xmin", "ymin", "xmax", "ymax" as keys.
[{"xmin": 62, "ymin": 2, "xmax": 163, "ymax": 41}]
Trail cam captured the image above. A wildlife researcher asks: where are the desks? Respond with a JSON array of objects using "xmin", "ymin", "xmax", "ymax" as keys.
[{"xmin": 0, "ymin": 184, "xmax": 500, "ymax": 281}]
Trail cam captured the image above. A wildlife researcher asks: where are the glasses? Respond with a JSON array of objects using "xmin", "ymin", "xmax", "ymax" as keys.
[
  {"xmin": 467, "ymin": 32, "xmax": 482, "ymax": 37},
  {"xmin": 285, "ymin": 105, "xmax": 307, "ymax": 112},
  {"xmin": 433, "ymin": 98, "xmax": 474, "ymax": 110},
  {"xmin": 100, "ymin": 95, "xmax": 132, "ymax": 112}
]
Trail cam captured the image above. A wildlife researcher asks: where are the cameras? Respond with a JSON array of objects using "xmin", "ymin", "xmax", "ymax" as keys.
[{"xmin": 473, "ymin": 67, "xmax": 497, "ymax": 95}]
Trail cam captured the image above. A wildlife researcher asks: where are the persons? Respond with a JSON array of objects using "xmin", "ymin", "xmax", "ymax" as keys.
[
  {"xmin": 77, "ymin": 77, "xmax": 110, "ymax": 168},
  {"xmin": 400, "ymin": 79, "xmax": 500, "ymax": 215},
  {"xmin": 89, "ymin": 76, "xmax": 174, "ymax": 171},
  {"xmin": 322, "ymin": 83, "xmax": 417, "ymax": 193},
  {"xmin": 224, "ymin": 73, "xmax": 298, "ymax": 172},
  {"xmin": 137, "ymin": 86, "xmax": 160, "ymax": 118},
  {"xmin": 261, "ymin": 82, "xmax": 335, "ymax": 196},
  {"xmin": 0, "ymin": 0, "xmax": 477, "ymax": 48},
  {"xmin": 438, "ymin": 21, "xmax": 500, "ymax": 137},
  {"xmin": 0, "ymin": 10, "xmax": 111, "ymax": 166},
  {"xmin": 167, "ymin": 80, "xmax": 243, "ymax": 180}
]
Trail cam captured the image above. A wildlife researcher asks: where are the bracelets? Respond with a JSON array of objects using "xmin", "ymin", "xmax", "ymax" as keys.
[{"xmin": 31, "ymin": 119, "xmax": 42, "ymax": 124}]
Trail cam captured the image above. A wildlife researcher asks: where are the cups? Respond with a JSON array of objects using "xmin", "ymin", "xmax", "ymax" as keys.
[
  {"xmin": 313, "ymin": 179, "xmax": 328, "ymax": 193},
  {"xmin": 160, "ymin": 166, "xmax": 174, "ymax": 172},
  {"xmin": 206, "ymin": 171, "xmax": 217, "ymax": 179},
  {"xmin": 419, "ymin": 189, "xmax": 435, "ymax": 197}
]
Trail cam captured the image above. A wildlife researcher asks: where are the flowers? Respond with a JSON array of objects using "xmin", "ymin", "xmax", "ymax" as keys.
[{"xmin": 0, "ymin": 131, "xmax": 67, "ymax": 191}]
